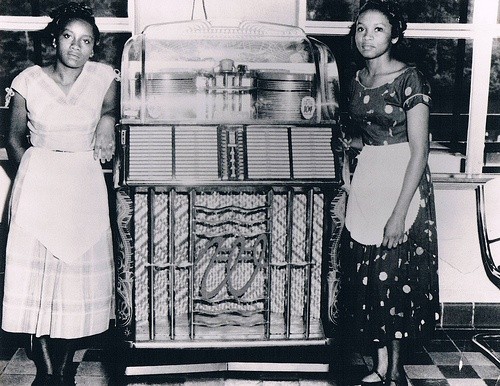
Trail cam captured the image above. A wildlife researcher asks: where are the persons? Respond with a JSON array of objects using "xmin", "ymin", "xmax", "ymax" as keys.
[
  {"xmin": 328, "ymin": 1, "xmax": 440, "ymax": 386},
  {"xmin": 0, "ymin": 2, "xmax": 120, "ymax": 386}
]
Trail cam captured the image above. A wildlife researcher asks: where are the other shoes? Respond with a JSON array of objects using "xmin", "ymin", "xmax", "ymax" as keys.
[{"xmin": 349, "ymin": 371, "xmax": 387, "ymax": 386}]
